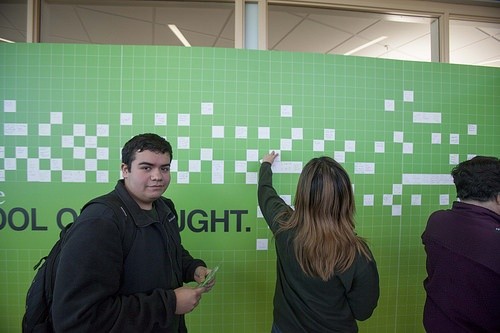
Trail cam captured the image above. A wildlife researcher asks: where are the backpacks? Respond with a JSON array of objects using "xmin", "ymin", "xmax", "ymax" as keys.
[{"xmin": 22, "ymin": 195, "xmax": 180, "ymax": 333}]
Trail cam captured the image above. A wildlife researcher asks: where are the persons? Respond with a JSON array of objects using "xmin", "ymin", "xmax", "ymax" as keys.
[
  {"xmin": 48, "ymin": 132, "xmax": 217, "ymax": 333},
  {"xmin": 258, "ymin": 151, "xmax": 380, "ymax": 333},
  {"xmin": 420, "ymin": 156, "xmax": 500, "ymax": 333}
]
